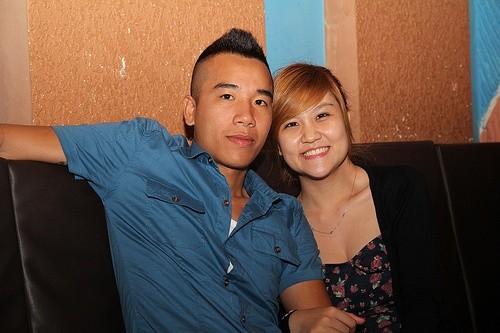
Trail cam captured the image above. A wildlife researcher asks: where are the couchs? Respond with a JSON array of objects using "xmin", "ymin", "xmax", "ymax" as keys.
[{"xmin": 0, "ymin": 139, "xmax": 500, "ymax": 333}]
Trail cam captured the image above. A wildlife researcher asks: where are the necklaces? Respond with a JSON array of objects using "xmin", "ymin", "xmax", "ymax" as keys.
[{"xmin": 305, "ymin": 163, "xmax": 359, "ymax": 239}]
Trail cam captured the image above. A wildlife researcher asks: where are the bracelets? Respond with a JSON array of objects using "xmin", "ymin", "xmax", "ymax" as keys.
[{"xmin": 279, "ymin": 309, "xmax": 299, "ymax": 333}]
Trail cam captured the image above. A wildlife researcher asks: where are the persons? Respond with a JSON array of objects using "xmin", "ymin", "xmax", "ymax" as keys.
[
  {"xmin": 1, "ymin": 26, "xmax": 336, "ymax": 333},
  {"xmin": 260, "ymin": 61, "xmax": 454, "ymax": 333}
]
